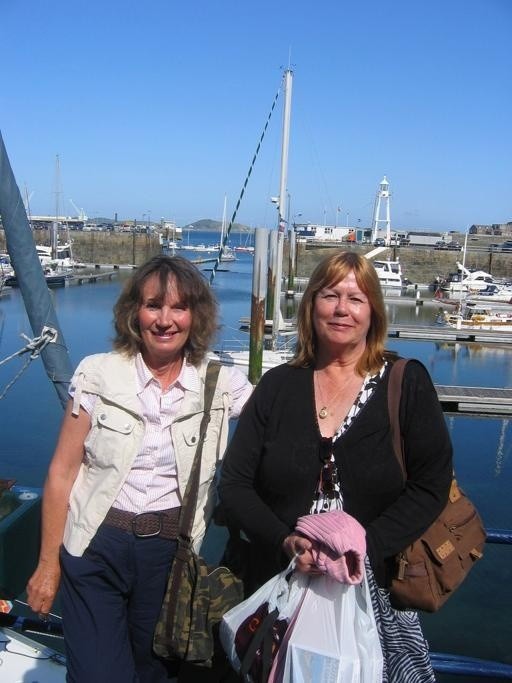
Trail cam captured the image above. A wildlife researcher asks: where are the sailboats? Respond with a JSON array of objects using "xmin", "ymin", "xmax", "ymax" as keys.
[{"xmin": 165, "ymin": 194, "xmax": 258, "ymax": 264}]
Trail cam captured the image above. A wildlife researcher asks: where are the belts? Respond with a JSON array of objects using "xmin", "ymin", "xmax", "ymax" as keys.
[{"xmin": 103, "ymin": 507, "xmax": 181, "ymax": 540}]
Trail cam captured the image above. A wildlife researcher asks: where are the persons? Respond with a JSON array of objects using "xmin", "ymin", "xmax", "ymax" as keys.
[
  {"xmin": 210, "ymin": 247, "xmax": 457, "ymax": 681},
  {"xmin": 23, "ymin": 254, "xmax": 260, "ymax": 682}
]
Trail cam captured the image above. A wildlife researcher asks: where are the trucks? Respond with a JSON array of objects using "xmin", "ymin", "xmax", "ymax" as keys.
[{"xmin": 395, "ymin": 231, "xmax": 444, "ymax": 245}]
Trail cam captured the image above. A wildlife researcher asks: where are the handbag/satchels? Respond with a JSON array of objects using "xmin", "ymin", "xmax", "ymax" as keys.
[
  {"xmin": 152, "ymin": 548, "xmax": 244, "ymax": 673},
  {"xmin": 391, "ymin": 479, "xmax": 488, "ymax": 613}
]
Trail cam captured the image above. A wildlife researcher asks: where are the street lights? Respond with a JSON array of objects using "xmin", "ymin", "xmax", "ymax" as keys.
[{"xmin": 293, "ymin": 212, "xmax": 304, "ymax": 277}]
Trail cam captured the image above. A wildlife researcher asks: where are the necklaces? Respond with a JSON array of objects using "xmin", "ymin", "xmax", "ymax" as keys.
[{"xmin": 315, "ymin": 367, "xmax": 352, "ymax": 419}]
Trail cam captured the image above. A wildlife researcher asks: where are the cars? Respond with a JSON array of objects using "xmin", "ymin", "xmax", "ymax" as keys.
[
  {"xmin": 30, "ymin": 220, "xmax": 154, "ymax": 233},
  {"xmin": 490, "ymin": 241, "xmax": 511, "ymax": 252},
  {"xmin": 374, "ymin": 236, "xmax": 411, "ymax": 248}
]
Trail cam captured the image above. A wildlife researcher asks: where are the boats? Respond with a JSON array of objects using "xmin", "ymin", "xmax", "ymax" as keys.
[
  {"xmin": 438, "ymin": 300, "xmax": 512, "ymax": 332},
  {"xmin": 363, "ymin": 253, "xmax": 405, "ymax": 286},
  {"xmin": 5, "ymin": 262, "xmax": 74, "ymax": 284},
  {"xmin": 433, "ymin": 262, "xmax": 512, "ymax": 303},
  {"xmin": 1, "ymin": 243, "xmax": 78, "ymax": 268}
]
